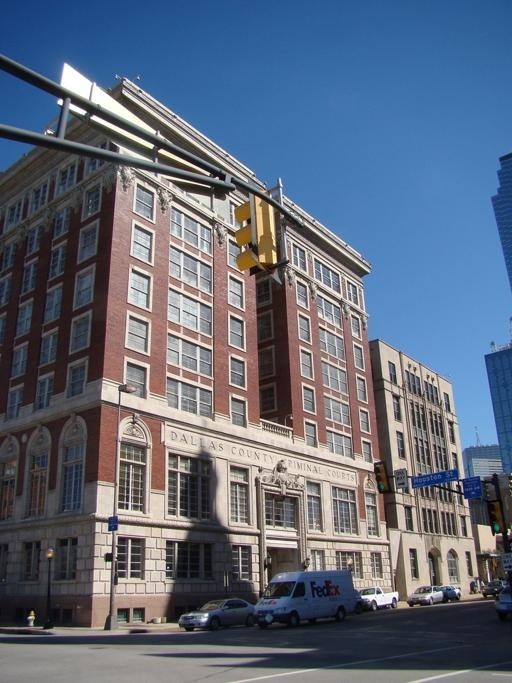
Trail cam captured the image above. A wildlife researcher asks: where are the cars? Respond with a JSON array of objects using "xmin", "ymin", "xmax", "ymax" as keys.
[
  {"xmin": 495, "ymin": 583, "xmax": 512, "ymax": 621},
  {"xmin": 407, "ymin": 585, "xmax": 461, "ymax": 607},
  {"xmin": 469, "ymin": 580, "xmax": 510, "ymax": 597},
  {"xmin": 178, "ymin": 597, "xmax": 257, "ymax": 631}
]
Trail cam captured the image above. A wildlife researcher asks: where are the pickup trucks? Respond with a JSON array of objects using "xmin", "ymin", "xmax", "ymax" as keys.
[{"xmin": 355, "ymin": 586, "xmax": 399, "ymax": 612}]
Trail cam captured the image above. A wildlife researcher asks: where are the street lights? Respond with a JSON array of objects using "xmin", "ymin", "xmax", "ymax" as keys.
[
  {"xmin": 104, "ymin": 384, "xmax": 138, "ymax": 630},
  {"xmin": 43, "ymin": 547, "xmax": 55, "ymax": 628}
]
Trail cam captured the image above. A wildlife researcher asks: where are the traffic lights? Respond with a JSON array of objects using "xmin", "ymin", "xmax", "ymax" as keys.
[
  {"xmin": 234, "ymin": 188, "xmax": 278, "ymax": 277},
  {"xmin": 508, "ymin": 475, "xmax": 512, "ymax": 496},
  {"xmin": 374, "ymin": 461, "xmax": 392, "ymax": 493},
  {"xmin": 487, "ymin": 501, "xmax": 505, "ymax": 536}
]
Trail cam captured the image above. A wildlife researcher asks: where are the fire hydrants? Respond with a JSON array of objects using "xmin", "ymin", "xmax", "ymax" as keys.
[{"xmin": 27, "ymin": 609, "xmax": 36, "ymax": 627}]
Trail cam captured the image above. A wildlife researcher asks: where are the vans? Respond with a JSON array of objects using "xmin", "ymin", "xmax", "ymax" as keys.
[{"xmin": 253, "ymin": 569, "xmax": 356, "ymax": 628}]
